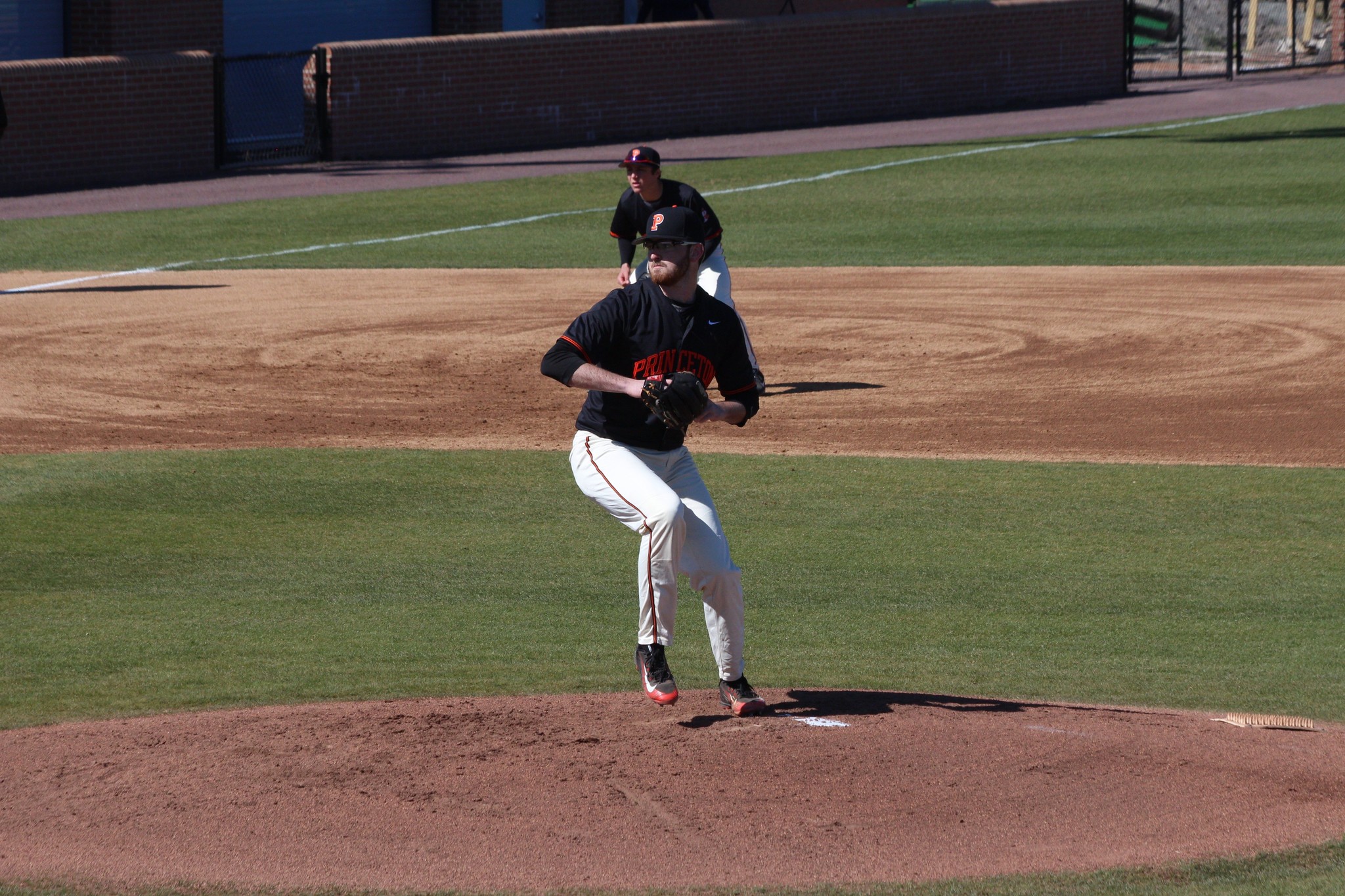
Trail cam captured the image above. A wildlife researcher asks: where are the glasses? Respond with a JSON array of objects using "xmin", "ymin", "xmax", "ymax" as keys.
[{"xmin": 642, "ymin": 239, "xmax": 701, "ymax": 253}]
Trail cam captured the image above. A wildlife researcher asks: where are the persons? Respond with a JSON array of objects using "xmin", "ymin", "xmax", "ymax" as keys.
[
  {"xmin": 541, "ymin": 205, "xmax": 767, "ymax": 715},
  {"xmin": 610, "ymin": 146, "xmax": 765, "ymax": 396}
]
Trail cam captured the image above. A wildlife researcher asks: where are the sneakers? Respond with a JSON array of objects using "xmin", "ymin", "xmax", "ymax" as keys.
[
  {"xmin": 753, "ymin": 367, "xmax": 766, "ymax": 397},
  {"xmin": 719, "ymin": 675, "xmax": 765, "ymax": 718},
  {"xmin": 634, "ymin": 639, "xmax": 678, "ymax": 708}
]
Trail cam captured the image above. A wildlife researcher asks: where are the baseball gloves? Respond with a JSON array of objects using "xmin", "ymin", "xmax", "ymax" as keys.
[{"xmin": 640, "ymin": 370, "xmax": 710, "ymax": 438}]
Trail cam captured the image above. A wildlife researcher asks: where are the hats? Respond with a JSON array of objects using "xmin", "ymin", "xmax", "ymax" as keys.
[
  {"xmin": 632, "ymin": 204, "xmax": 705, "ymax": 246},
  {"xmin": 618, "ymin": 145, "xmax": 660, "ymax": 168}
]
